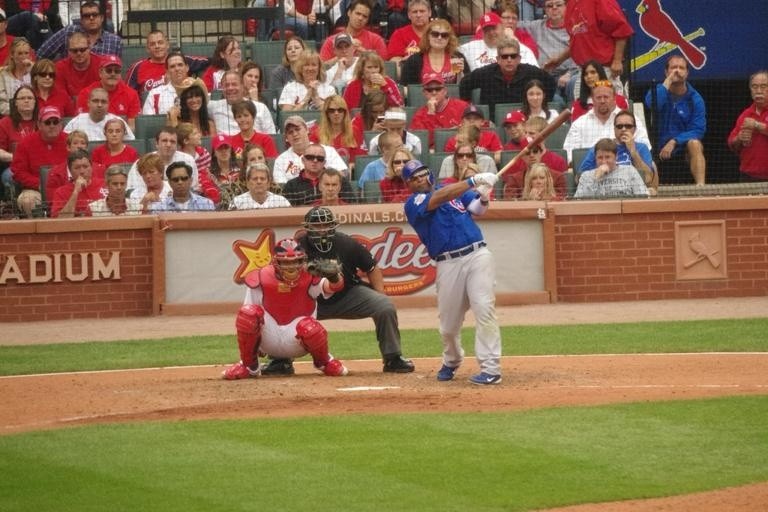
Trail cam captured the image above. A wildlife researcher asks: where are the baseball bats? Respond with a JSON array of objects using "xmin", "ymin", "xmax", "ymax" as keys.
[{"xmin": 475, "ymin": 108, "xmax": 572, "ymax": 200}]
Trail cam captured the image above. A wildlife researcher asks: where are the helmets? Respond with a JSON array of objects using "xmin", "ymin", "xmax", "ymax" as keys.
[
  {"xmin": 271, "ymin": 238, "xmax": 308, "ymax": 286},
  {"xmin": 401, "ymin": 160, "xmax": 435, "ymax": 185},
  {"xmin": 299, "ymin": 205, "xmax": 340, "ymax": 254}
]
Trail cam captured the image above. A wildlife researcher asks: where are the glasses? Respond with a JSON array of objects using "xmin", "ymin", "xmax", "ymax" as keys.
[
  {"xmin": 304, "ymin": 154, "xmax": 324, "ymax": 162},
  {"xmin": 392, "ymin": 159, "xmax": 410, "ymax": 165},
  {"xmin": 328, "ymin": 107, "xmax": 346, "ymax": 113},
  {"xmin": 545, "ymin": 1, "xmax": 562, "ymax": 8},
  {"xmin": 430, "ymin": 31, "xmax": 448, "ymax": 38},
  {"xmin": 424, "ymin": 87, "xmax": 443, "ymax": 92},
  {"xmin": 616, "ymin": 123, "xmax": 635, "ymax": 130},
  {"xmin": 500, "ymin": 54, "xmax": 517, "ymax": 59},
  {"xmin": 172, "ymin": 176, "xmax": 187, "ymax": 182},
  {"xmin": 15, "ymin": 12, "xmax": 122, "ymax": 126},
  {"xmin": 455, "ymin": 153, "xmax": 474, "ymax": 160},
  {"xmin": 524, "ymin": 147, "xmax": 538, "ymax": 156}
]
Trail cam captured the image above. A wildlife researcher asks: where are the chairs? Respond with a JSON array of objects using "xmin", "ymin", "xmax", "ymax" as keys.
[{"xmin": 0, "ymin": 29, "xmax": 655, "ymax": 220}]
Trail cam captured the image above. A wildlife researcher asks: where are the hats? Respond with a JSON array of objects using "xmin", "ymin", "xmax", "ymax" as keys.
[
  {"xmin": 478, "ymin": 12, "xmax": 502, "ymax": 29},
  {"xmin": 422, "ymin": 72, "xmax": 445, "ymax": 85},
  {"xmin": 284, "ymin": 115, "xmax": 308, "ymax": 131},
  {"xmin": 518, "ymin": 134, "xmax": 541, "ymax": 148},
  {"xmin": 210, "ymin": 132, "xmax": 231, "ymax": 151},
  {"xmin": 500, "ymin": 110, "xmax": 527, "ymax": 125},
  {"xmin": 173, "ymin": 75, "xmax": 208, "ymax": 97},
  {"xmin": 334, "ymin": 33, "xmax": 352, "ymax": 48},
  {"xmin": 462, "ymin": 106, "xmax": 484, "ymax": 119}
]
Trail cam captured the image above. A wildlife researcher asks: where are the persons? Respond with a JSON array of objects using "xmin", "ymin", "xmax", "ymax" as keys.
[
  {"xmin": 217, "ymin": 236, "xmax": 350, "ymax": 382},
  {"xmin": 646, "ymin": 55, "xmax": 706, "ymax": 186},
  {"xmin": 402, "ymin": 158, "xmax": 505, "ymax": 386},
  {"xmin": 727, "ymin": 70, "xmax": 768, "ymax": 184},
  {"xmin": 259, "ymin": 204, "xmax": 416, "ymax": 378},
  {"xmin": 1, "ymin": 1, "xmax": 292, "ymax": 221},
  {"xmin": 246, "ymin": 1, "xmax": 659, "ymax": 210}
]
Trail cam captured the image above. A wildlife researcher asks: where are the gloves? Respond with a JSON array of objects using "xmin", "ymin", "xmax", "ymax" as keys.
[
  {"xmin": 476, "ymin": 184, "xmax": 490, "ymax": 201},
  {"xmin": 470, "ymin": 172, "xmax": 500, "ymax": 187}
]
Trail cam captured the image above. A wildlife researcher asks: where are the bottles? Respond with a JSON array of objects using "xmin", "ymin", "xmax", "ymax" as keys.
[{"xmin": 741, "ymin": 118, "xmax": 752, "ymax": 147}]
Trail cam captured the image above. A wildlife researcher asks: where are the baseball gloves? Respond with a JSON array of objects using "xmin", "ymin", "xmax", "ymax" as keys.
[{"xmin": 309, "ymin": 259, "xmax": 342, "ymax": 283}]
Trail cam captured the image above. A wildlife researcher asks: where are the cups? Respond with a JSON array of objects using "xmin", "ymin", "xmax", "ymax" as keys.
[
  {"xmin": 371, "ymin": 74, "xmax": 381, "ymax": 89},
  {"xmin": 451, "ymin": 57, "xmax": 461, "ymax": 74}
]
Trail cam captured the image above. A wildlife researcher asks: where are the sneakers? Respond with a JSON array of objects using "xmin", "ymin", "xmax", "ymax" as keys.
[
  {"xmin": 437, "ymin": 363, "xmax": 459, "ymax": 381},
  {"xmin": 468, "ymin": 371, "xmax": 502, "ymax": 385},
  {"xmin": 313, "ymin": 352, "xmax": 349, "ymax": 376},
  {"xmin": 382, "ymin": 355, "xmax": 415, "ymax": 372},
  {"xmin": 222, "ymin": 359, "xmax": 262, "ymax": 379},
  {"xmin": 261, "ymin": 359, "xmax": 295, "ymax": 375}
]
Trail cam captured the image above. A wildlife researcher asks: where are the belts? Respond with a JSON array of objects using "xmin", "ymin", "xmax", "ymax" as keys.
[{"xmin": 434, "ymin": 241, "xmax": 484, "ymax": 261}]
